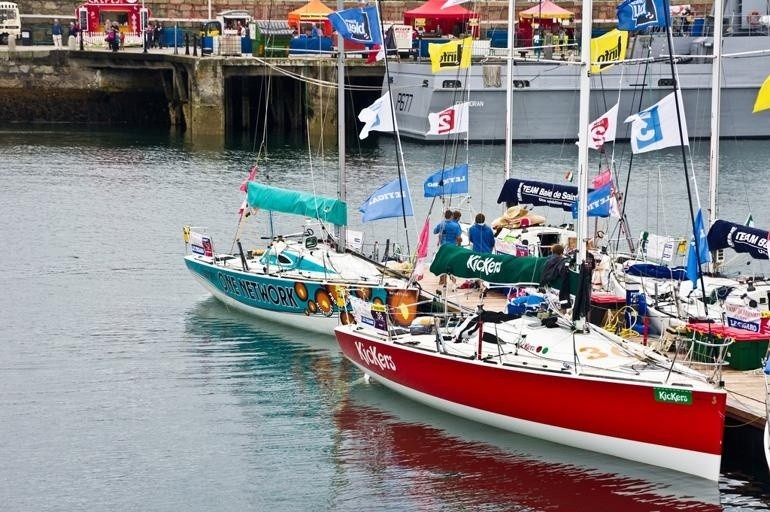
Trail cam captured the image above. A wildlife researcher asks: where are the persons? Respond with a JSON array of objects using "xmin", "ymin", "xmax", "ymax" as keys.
[
  {"xmin": 66, "ymin": 20, "xmax": 81, "ymax": 49},
  {"xmin": 119, "ymin": 32, "xmax": 125, "ymax": 51},
  {"xmin": 109, "ymin": 21, "xmax": 119, "ymax": 52},
  {"xmin": 469, "ymin": 213, "xmax": 495, "ymax": 298},
  {"xmin": 533, "ymin": 31, "xmax": 541, "ymax": 55},
  {"xmin": 558, "ymin": 26, "xmax": 567, "ymax": 51},
  {"xmin": 146, "ymin": 23, "xmax": 153, "ymax": 49},
  {"xmin": 432, "ymin": 210, "xmax": 462, "ymax": 285},
  {"xmin": 317, "ymin": 24, "xmax": 324, "ymax": 38},
  {"xmin": 225, "ymin": 21, "xmax": 249, "ymax": 37},
  {"xmin": 451, "ymin": 21, "xmax": 461, "ymax": 39},
  {"xmin": 152, "ymin": 20, "xmax": 163, "ymax": 50},
  {"xmin": 537, "ymin": 245, "xmax": 572, "ymax": 315},
  {"xmin": 438, "ymin": 211, "xmax": 461, "ymax": 284},
  {"xmin": 51, "ymin": 18, "xmax": 65, "ymax": 48}
]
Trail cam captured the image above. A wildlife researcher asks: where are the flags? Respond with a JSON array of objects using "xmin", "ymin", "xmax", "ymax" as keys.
[
  {"xmin": 590, "ymin": 169, "xmax": 623, "ymax": 219},
  {"xmin": 423, "ymin": 163, "xmax": 470, "ymax": 199},
  {"xmin": 428, "ymin": 37, "xmax": 472, "ymax": 76},
  {"xmin": 327, "ymin": 5, "xmax": 383, "ymax": 46},
  {"xmin": 623, "ymin": 90, "xmax": 690, "ymax": 156},
  {"xmin": 426, "ymin": 102, "xmax": 467, "ymax": 137},
  {"xmin": 575, "ymin": 104, "xmax": 618, "ymax": 151},
  {"xmin": 686, "ymin": 209, "xmax": 711, "ymax": 290},
  {"xmin": 613, "ymin": 1, "xmax": 673, "ymax": 32},
  {"xmin": 359, "ymin": 176, "xmax": 413, "ymax": 226},
  {"xmin": 590, "ymin": 24, "xmax": 629, "ymax": 76},
  {"xmin": 570, "ymin": 182, "xmax": 610, "ymax": 220},
  {"xmin": 358, "ymin": 91, "xmax": 398, "ymax": 143}
]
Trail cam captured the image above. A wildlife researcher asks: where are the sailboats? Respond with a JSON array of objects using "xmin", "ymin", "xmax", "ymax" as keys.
[{"xmin": 183, "ymin": 0, "xmax": 421, "ymax": 337}]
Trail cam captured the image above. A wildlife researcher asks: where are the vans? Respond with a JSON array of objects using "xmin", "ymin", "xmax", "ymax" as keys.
[{"xmin": 0, "ymin": 1, "xmax": 22, "ymax": 45}]
